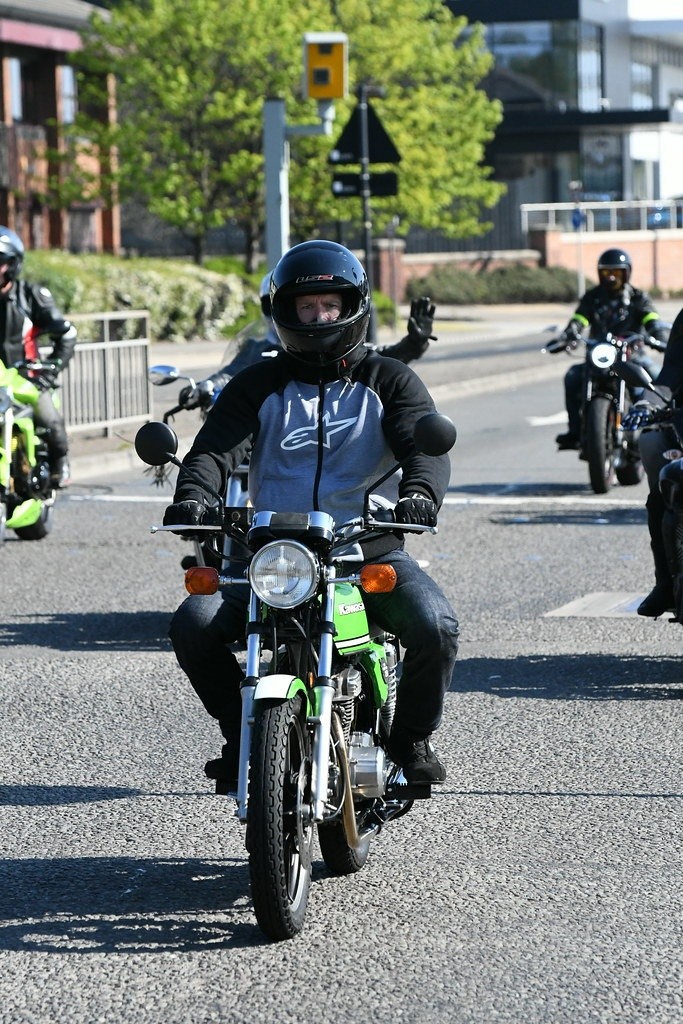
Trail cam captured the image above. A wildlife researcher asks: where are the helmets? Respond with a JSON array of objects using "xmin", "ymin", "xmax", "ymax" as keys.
[
  {"xmin": 0, "ymin": 226, "xmax": 25, "ymax": 282},
  {"xmin": 259, "ymin": 268, "xmax": 272, "ymax": 317},
  {"xmin": 597, "ymin": 249, "xmax": 632, "ymax": 292},
  {"xmin": 269, "ymin": 240, "xmax": 370, "ymax": 366}
]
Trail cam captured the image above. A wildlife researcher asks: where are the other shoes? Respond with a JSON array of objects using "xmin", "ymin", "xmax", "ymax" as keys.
[
  {"xmin": 47, "ymin": 456, "xmax": 70, "ymax": 490},
  {"xmin": 556, "ymin": 422, "xmax": 583, "ymax": 445}
]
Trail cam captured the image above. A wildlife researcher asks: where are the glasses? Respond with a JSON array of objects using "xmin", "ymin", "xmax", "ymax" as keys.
[{"xmin": 600, "ymin": 269, "xmax": 623, "ymax": 278}]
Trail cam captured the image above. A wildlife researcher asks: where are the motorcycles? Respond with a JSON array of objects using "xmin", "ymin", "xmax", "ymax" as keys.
[
  {"xmin": 539, "ymin": 323, "xmax": 676, "ymax": 494},
  {"xmin": 148, "ymin": 366, "xmax": 255, "ymax": 578},
  {"xmin": 135, "ymin": 410, "xmax": 459, "ymax": 943},
  {"xmin": 611, "ymin": 357, "xmax": 683, "ymax": 628},
  {"xmin": 0, "ymin": 318, "xmax": 74, "ymax": 541}
]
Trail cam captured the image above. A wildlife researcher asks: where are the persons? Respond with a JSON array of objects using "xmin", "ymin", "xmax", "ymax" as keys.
[
  {"xmin": 0, "ymin": 226, "xmax": 78, "ymax": 488},
  {"xmin": 547, "ymin": 249, "xmax": 668, "ymax": 444},
  {"xmin": 163, "ymin": 242, "xmax": 460, "ymax": 785},
  {"xmin": 180, "ymin": 269, "xmax": 438, "ymax": 569},
  {"xmin": 620, "ymin": 310, "xmax": 683, "ymax": 616}
]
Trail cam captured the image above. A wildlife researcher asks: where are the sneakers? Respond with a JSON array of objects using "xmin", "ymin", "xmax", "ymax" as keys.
[
  {"xmin": 388, "ymin": 725, "xmax": 446, "ymax": 784},
  {"xmin": 204, "ymin": 758, "xmax": 238, "ymax": 779}
]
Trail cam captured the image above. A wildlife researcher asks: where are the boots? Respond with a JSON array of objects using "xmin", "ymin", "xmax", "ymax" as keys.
[{"xmin": 638, "ymin": 543, "xmax": 676, "ymax": 617}]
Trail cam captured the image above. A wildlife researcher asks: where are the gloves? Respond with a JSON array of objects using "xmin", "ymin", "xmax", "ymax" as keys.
[
  {"xmin": 163, "ymin": 500, "xmax": 209, "ymax": 537},
  {"xmin": 546, "ymin": 339, "xmax": 568, "ymax": 355},
  {"xmin": 393, "ymin": 492, "xmax": 438, "ymax": 540},
  {"xmin": 179, "ymin": 379, "xmax": 215, "ymax": 410},
  {"xmin": 656, "ymin": 329, "xmax": 671, "ymax": 353},
  {"xmin": 407, "ymin": 296, "xmax": 438, "ymax": 359},
  {"xmin": 27, "ymin": 371, "xmax": 60, "ymax": 395}
]
